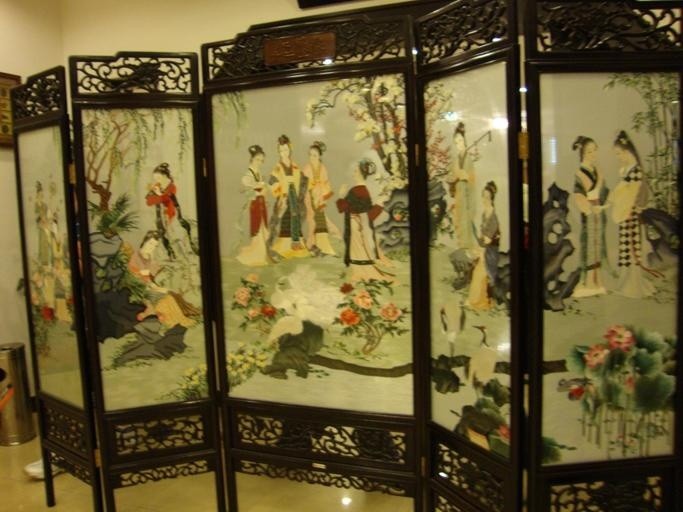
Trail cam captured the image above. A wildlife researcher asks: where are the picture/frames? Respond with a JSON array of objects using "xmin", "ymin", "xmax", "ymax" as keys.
[{"xmin": 0, "ymin": 71, "xmax": 23, "ymax": 148}]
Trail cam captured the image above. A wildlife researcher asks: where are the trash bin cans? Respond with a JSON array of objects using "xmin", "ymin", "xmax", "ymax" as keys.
[{"xmin": 0, "ymin": 342, "xmax": 35, "ymax": 447}]
